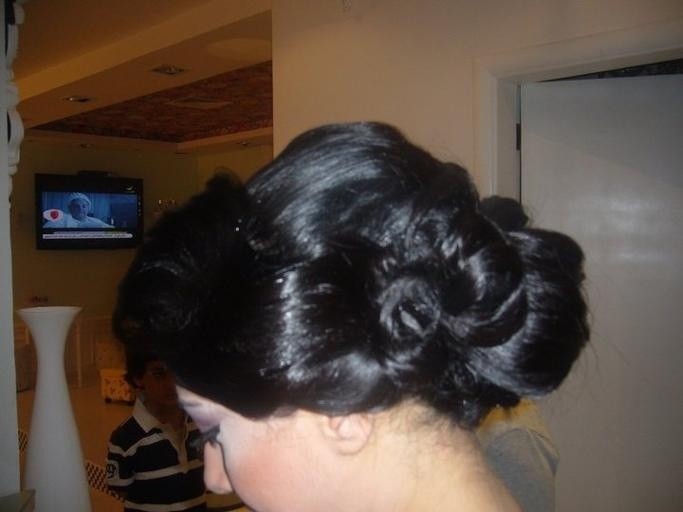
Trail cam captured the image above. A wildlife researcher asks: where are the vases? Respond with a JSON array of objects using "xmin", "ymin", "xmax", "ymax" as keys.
[{"xmin": 17, "ymin": 306, "xmax": 91, "ymax": 512}]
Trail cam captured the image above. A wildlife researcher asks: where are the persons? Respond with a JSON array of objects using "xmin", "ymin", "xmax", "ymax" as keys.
[
  {"xmin": 42, "ymin": 193, "xmax": 114, "ymax": 228},
  {"xmin": 472, "ymin": 395, "xmax": 564, "ymax": 511},
  {"xmin": 102, "ymin": 338, "xmax": 210, "ymax": 512},
  {"xmin": 105, "ymin": 118, "xmax": 593, "ymax": 512}
]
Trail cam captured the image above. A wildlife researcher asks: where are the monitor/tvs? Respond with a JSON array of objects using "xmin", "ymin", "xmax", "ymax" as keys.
[{"xmin": 31, "ymin": 172, "xmax": 145, "ymax": 251}]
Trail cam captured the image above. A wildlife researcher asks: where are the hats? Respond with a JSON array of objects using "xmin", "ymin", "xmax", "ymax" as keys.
[{"xmin": 67, "ymin": 192, "xmax": 89, "ymax": 208}]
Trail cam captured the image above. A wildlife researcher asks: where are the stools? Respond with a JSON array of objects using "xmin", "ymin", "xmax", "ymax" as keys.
[{"xmin": 101, "ymin": 368, "xmax": 136, "ymax": 405}]
